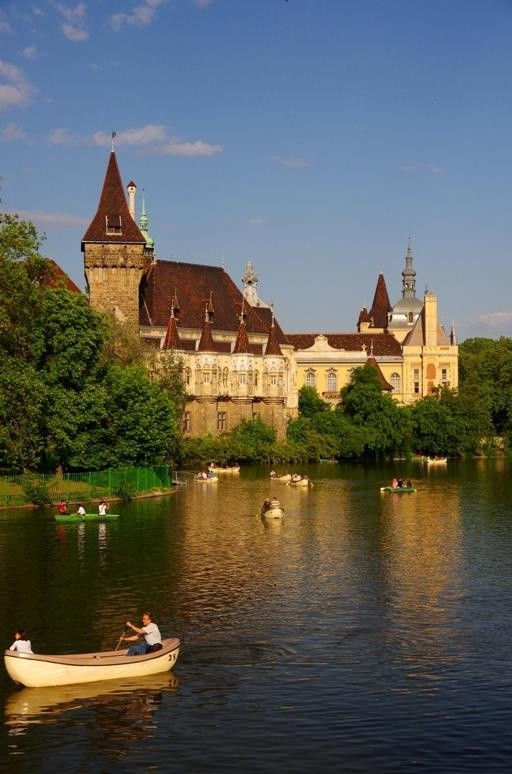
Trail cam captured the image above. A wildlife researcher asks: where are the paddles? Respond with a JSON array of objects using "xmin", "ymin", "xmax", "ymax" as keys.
[
  {"xmin": 404, "ymin": 483, "xmax": 417, "ymax": 492},
  {"xmin": 380, "ymin": 487, "xmax": 391, "ymax": 490}
]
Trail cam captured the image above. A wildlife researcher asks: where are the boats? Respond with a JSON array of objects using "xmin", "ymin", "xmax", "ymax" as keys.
[
  {"xmin": 207, "ymin": 465, "xmax": 240, "ymax": 472},
  {"xmin": 2, "ymin": 636, "xmax": 182, "ymax": 689},
  {"xmin": 425, "ymin": 456, "xmax": 448, "ymax": 464},
  {"xmin": 270, "ymin": 472, "xmax": 311, "ymax": 486},
  {"xmin": 195, "ymin": 476, "xmax": 219, "ymax": 483},
  {"xmin": 260, "ymin": 506, "xmax": 285, "ymax": 520},
  {"xmin": 379, "ymin": 486, "xmax": 417, "ymax": 493},
  {"xmin": 54, "ymin": 513, "xmax": 121, "ymax": 522}
]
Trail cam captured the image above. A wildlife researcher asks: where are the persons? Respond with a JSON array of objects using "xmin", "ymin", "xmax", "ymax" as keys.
[
  {"xmin": 9, "ymin": 629, "xmax": 34, "ymax": 654},
  {"xmin": 76, "ymin": 503, "xmax": 87, "ymax": 516},
  {"xmin": 57, "ymin": 499, "xmax": 70, "ymax": 515},
  {"xmin": 392, "ymin": 478, "xmax": 412, "ymax": 489},
  {"xmin": 269, "ymin": 468, "xmax": 276, "ymax": 477},
  {"xmin": 196, "ymin": 462, "xmax": 239, "ymax": 480},
  {"xmin": 98, "ymin": 501, "xmax": 111, "ymax": 515},
  {"xmin": 290, "ymin": 472, "xmax": 310, "ymax": 482},
  {"xmin": 118, "ymin": 610, "xmax": 163, "ymax": 655},
  {"xmin": 262, "ymin": 497, "xmax": 272, "ymax": 513},
  {"xmin": 271, "ymin": 495, "xmax": 285, "ymax": 511}
]
